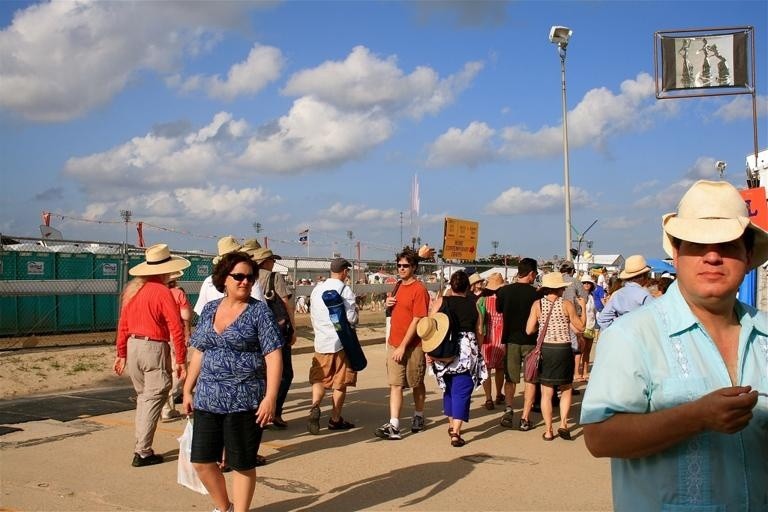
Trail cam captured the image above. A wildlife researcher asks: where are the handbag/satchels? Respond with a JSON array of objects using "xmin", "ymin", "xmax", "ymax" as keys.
[
  {"xmin": 524, "ymin": 346, "xmax": 543, "ymax": 384},
  {"xmin": 263, "ymin": 290, "xmax": 293, "ymax": 346},
  {"xmin": 427, "ymin": 300, "xmax": 459, "ymax": 358},
  {"xmin": 476, "ymin": 303, "xmax": 488, "ymax": 338}
]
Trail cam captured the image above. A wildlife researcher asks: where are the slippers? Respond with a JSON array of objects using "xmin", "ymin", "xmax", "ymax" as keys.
[
  {"xmin": 557, "ymin": 427, "xmax": 572, "ymax": 441},
  {"xmin": 542, "ymin": 432, "xmax": 555, "ymax": 441}
]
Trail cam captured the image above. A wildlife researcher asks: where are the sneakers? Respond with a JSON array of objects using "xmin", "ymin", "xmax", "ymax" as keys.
[
  {"xmin": 130, "ymin": 451, "xmax": 163, "ymax": 467},
  {"xmin": 500, "ymin": 411, "xmax": 514, "ymax": 429},
  {"xmin": 269, "ymin": 416, "xmax": 289, "ymax": 430},
  {"xmin": 374, "ymin": 422, "xmax": 403, "ymax": 440},
  {"xmin": 572, "ymin": 373, "xmax": 591, "ymax": 383},
  {"xmin": 411, "ymin": 415, "xmax": 426, "ymax": 433},
  {"xmin": 256, "ymin": 455, "xmax": 266, "ymax": 466},
  {"xmin": 217, "ymin": 459, "xmax": 234, "ymax": 473},
  {"xmin": 213, "ymin": 502, "xmax": 234, "ymax": 512},
  {"xmin": 485, "ymin": 393, "xmax": 506, "ymax": 410},
  {"xmin": 518, "ymin": 419, "xmax": 536, "ymax": 432},
  {"xmin": 306, "ymin": 403, "xmax": 321, "ymax": 435},
  {"xmin": 161, "ymin": 409, "xmax": 180, "ymax": 420}
]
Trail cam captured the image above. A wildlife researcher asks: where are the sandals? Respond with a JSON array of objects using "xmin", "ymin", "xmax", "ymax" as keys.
[
  {"xmin": 327, "ymin": 416, "xmax": 356, "ymax": 430},
  {"xmin": 447, "ymin": 425, "xmax": 465, "ymax": 447}
]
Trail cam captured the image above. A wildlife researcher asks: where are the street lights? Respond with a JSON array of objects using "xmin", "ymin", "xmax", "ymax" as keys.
[
  {"xmin": 399, "ymin": 211, "xmax": 421, "ymax": 253},
  {"xmin": 119, "ymin": 208, "xmax": 133, "ymax": 250},
  {"xmin": 346, "ymin": 230, "xmax": 354, "ymax": 258},
  {"xmin": 491, "ymin": 240, "xmax": 500, "ymax": 253},
  {"xmin": 715, "ymin": 158, "xmax": 727, "ymax": 179},
  {"xmin": 251, "ymin": 221, "xmax": 263, "ymax": 238},
  {"xmin": 547, "ymin": 24, "xmax": 576, "ymax": 262}
]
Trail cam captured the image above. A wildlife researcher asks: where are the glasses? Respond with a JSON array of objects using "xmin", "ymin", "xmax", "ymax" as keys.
[
  {"xmin": 228, "ymin": 273, "xmax": 255, "ymax": 283},
  {"xmin": 398, "ymin": 263, "xmax": 412, "ymax": 268}
]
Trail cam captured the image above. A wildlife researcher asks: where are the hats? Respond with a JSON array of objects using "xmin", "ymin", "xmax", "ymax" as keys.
[
  {"xmin": 163, "ymin": 270, "xmax": 184, "ymax": 283},
  {"xmin": 127, "ymin": 243, "xmax": 192, "ymax": 277},
  {"xmin": 618, "ymin": 255, "xmax": 651, "ymax": 280},
  {"xmin": 661, "ymin": 180, "xmax": 768, "ymax": 270},
  {"xmin": 243, "ymin": 238, "xmax": 282, "ymax": 265},
  {"xmin": 416, "ymin": 311, "xmax": 450, "ymax": 353},
  {"xmin": 484, "ymin": 272, "xmax": 510, "ymax": 290},
  {"xmin": 468, "ymin": 272, "xmax": 486, "ymax": 286},
  {"xmin": 580, "ymin": 275, "xmax": 597, "ymax": 290},
  {"xmin": 559, "ymin": 260, "xmax": 576, "ymax": 271},
  {"xmin": 330, "ymin": 257, "xmax": 352, "ymax": 270},
  {"xmin": 535, "ymin": 272, "xmax": 573, "ymax": 292},
  {"xmin": 212, "ymin": 236, "xmax": 252, "ymax": 265}
]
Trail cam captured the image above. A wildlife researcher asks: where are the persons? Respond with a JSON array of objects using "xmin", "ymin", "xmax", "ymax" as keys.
[
  {"xmin": 159, "ymin": 270, "xmax": 193, "ymax": 422},
  {"xmin": 192, "ymin": 236, "xmax": 267, "ymax": 472},
  {"xmin": 112, "ymin": 243, "xmax": 189, "ymax": 467},
  {"xmin": 578, "ymin": 179, "xmax": 767, "ymax": 512},
  {"xmin": 192, "ymin": 239, "xmax": 676, "ymax": 450},
  {"xmin": 181, "ymin": 251, "xmax": 286, "ymax": 510}
]
